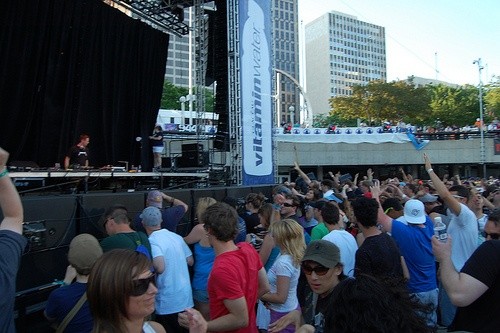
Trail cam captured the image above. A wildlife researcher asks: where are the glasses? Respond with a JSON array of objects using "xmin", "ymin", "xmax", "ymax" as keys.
[
  {"xmin": 103, "ymin": 217, "xmax": 114, "ymax": 227},
  {"xmin": 127, "ymin": 270, "xmax": 157, "ymax": 297},
  {"xmin": 245, "ymin": 200, "xmax": 249, "ymax": 204},
  {"xmin": 280, "ymin": 202, "xmax": 293, "ymax": 207},
  {"xmin": 481, "ymin": 231, "xmax": 499, "ymax": 239},
  {"xmin": 302, "ymin": 262, "xmax": 330, "ymax": 275}
]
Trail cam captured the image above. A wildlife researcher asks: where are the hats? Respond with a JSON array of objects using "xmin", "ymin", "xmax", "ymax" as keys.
[
  {"xmin": 147, "ymin": 189, "xmax": 162, "ymax": 208},
  {"xmin": 325, "ymin": 193, "xmax": 343, "ymax": 203},
  {"xmin": 310, "ymin": 200, "xmax": 327, "ymax": 208},
  {"xmin": 404, "ymin": 199, "xmax": 427, "ymax": 224},
  {"xmin": 418, "ymin": 191, "xmax": 438, "ymax": 202},
  {"xmin": 301, "ymin": 240, "xmax": 340, "ymax": 268},
  {"xmin": 68, "ymin": 233, "xmax": 103, "ymax": 268},
  {"xmin": 139, "ymin": 206, "xmax": 163, "ymax": 226}
]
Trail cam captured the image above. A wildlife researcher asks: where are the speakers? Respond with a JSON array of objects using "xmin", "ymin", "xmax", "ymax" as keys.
[{"xmin": 182, "ymin": 143, "xmax": 204, "ymax": 167}]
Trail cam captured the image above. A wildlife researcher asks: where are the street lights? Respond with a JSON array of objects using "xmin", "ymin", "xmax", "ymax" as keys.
[{"xmin": 473, "ymin": 58, "xmax": 484, "ymax": 179}]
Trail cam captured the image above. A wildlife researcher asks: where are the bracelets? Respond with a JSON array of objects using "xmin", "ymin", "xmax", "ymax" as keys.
[{"xmin": 0, "ymin": 167, "xmax": 8, "ymax": 178}]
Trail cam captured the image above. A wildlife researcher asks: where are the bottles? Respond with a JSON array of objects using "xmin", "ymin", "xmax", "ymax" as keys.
[
  {"xmin": 434, "ymin": 217, "xmax": 448, "ymax": 262},
  {"xmin": 138, "ymin": 165, "xmax": 142, "ymax": 172}
]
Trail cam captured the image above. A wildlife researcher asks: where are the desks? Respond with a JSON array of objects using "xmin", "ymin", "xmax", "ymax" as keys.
[{"xmin": 9, "ymin": 167, "xmax": 208, "ymax": 194}]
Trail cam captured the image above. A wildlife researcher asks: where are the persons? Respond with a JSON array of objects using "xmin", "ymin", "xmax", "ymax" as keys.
[
  {"xmin": 0, "ymin": 151, "xmax": 27, "ymax": 333},
  {"xmin": 46, "ymin": 153, "xmax": 500, "ymax": 333},
  {"xmin": 64, "ymin": 135, "xmax": 91, "ymax": 171},
  {"xmin": 417, "ymin": 116, "xmax": 500, "ymax": 136}
]
